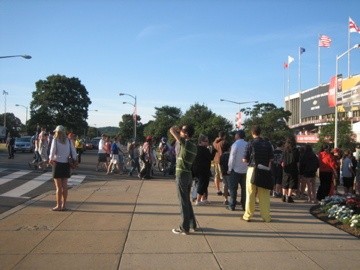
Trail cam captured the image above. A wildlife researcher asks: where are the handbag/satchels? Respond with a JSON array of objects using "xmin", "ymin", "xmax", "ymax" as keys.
[
  {"xmin": 68, "ymin": 155, "xmax": 75, "ymax": 170},
  {"xmin": 251, "ymin": 167, "xmax": 273, "ymax": 189}
]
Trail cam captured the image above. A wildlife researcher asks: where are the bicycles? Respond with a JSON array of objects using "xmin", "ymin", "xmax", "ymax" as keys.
[{"xmin": 157, "ymin": 152, "xmax": 169, "ymax": 173}]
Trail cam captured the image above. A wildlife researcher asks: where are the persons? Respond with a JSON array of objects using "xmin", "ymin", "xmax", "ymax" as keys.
[
  {"xmin": 49, "ymin": 124, "xmax": 78, "ymax": 211},
  {"xmin": 243, "ymin": 125, "xmax": 275, "ymax": 221},
  {"xmin": 193, "ymin": 129, "xmax": 250, "ymax": 210},
  {"xmin": 170, "ymin": 124, "xmax": 198, "ymax": 236},
  {"xmin": 270, "ymin": 136, "xmax": 360, "ymax": 203},
  {"xmin": 97, "ymin": 133, "xmax": 176, "ymax": 179},
  {"xmin": 28, "ymin": 126, "xmax": 83, "ymax": 169},
  {"xmin": 6, "ymin": 136, "xmax": 15, "ymax": 159}
]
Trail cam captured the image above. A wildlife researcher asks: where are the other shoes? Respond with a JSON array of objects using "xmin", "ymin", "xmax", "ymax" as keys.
[
  {"xmin": 217, "ymin": 191, "xmax": 222, "ymax": 195},
  {"xmin": 202, "ymin": 199, "xmax": 210, "ymax": 205},
  {"xmin": 172, "ymin": 229, "xmax": 187, "ymax": 235},
  {"xmin": 189, "ymin": 227, "xmax": 197, "ymax": 232},
  {"xmin": 275, "ymin": 193, "xmax": 282, "ymax": 197},
  {"xmin": 28, "ymin": 162, "xmax": 51, "ymax": 172},
  {"xmin": 96, "ymin": 167, "xmax": 176, "ymax": 179},
  {"xmin": 283, "ymin": 198, "xmax": 285, "ymax": 202},
  {"xmin": 224, "ymin": 201, "xmax": 228, "ymax": 206},
  {"xmin": 226, "ymin": 205, "xmax": 235, "ymax": 211},
  {"xmin": 194, "ymin": 201, "xmax": 206, "ymax": 206},
  {"xmin": 52, "ymin": 206, "xmax": 65, "ymax": 211},
  {"xmin": 241, "ymin": 215, "xmax": 250, "ymax": 222},
  {"xmin": 287, "ymin": 198, "xmax": 294, "ymax": 203}
]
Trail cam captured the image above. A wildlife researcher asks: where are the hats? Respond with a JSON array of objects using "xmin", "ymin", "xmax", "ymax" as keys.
[{"xmin": 53, "ymin": 125, "xmax": 66, "ymax": 133}]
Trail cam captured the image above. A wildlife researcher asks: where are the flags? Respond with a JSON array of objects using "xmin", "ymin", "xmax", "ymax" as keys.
[
  {"xmin": 328, "ymin": 77, "xmax": 336, "ymax": 107},
  {"xmin": 349, "ymin": 18, "xmax": 360, "ymax": 32},
  {"xmin": 318, "ymin": 35, "xmax": 332, "ymax": 47},
  {"xmin": 284, "ymin": 56, "xmax": 294, "ymax": 68},
  {"xmin": 300, "ymin": 47, "xmax": 305, "ymax": 55}
]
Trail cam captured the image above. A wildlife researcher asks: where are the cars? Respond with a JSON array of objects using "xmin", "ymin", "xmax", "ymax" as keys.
[
  {"xmin": 12, "ymin": 135, "xmax": 34, "ymax": 153},
  {"xmin": 84, "ymin": 136, "xmax": 101, "ymax": 150}
]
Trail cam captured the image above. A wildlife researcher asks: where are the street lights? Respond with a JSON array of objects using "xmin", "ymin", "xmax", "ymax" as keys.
[
  {"xmin": 220, "ymin": 98, "xmax": 258, "ymax": 131},
  {"xmin": 119, "ymin": 92, "xmax": 137, "ymax": 139},
  {"xmin": 15, "ymin": 103, "xmax": 27, "ymax": 135}
]
